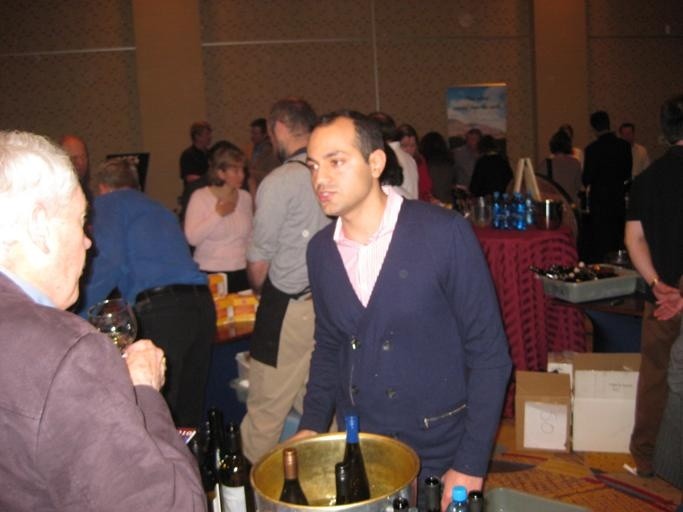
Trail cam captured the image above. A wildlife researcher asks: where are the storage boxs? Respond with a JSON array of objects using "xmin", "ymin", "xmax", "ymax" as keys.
[
  {"xmin": 547, "ymin": 352, "xmax": 575, "ymax": 389},
  {"xmin": 572, "ymin": 351, "xmax": 643, "ymax": 453},
  {"xmin": 514, "ymin": 371, "xmax": 570, "ymax": 453}
]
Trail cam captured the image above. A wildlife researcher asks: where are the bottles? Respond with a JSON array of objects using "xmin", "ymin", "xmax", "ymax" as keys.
[
  {"xmin": 530, "ymin": 264, "xmax": 546, "ymax": 276},
  {"xmin": 446, "ymin": 488, "xmax": 470, "ymax": 511},
  {"xmin": 345, "ymin": 416, "xmax": 368, "ymax": 505},
  {"xmin": 394, "ymin": 498, "xmax": 408, "ymax": 511},
  {"xmin": 547, "ymin": 263, "xmax": 618, "ymax": 284},
  {"xmin": 503, "ymin": 193, "xmax": 510, "ymax": 227},
  {"xmin": 537, "ymin": 197, "xmax": 560, "ymax": 231},
  {"xmin": 511, "ymin": 192, "xmax": 525, "ymax": 229},
  {"xmin": 334, "ymin": 462, "xmax": 352, "ymax": 507},
  {"xmin": 477, "ymin": 196, "xmax": 486, "ymax": 224},
  {"xmin": 493, "ymin": 193, "xmax": 500, "ymax": 227},
  {"xmin": 469, "ymin": 491, "xmax": 484, "ymax": 510},
  {"xmin": 525, "ymin": 190, "xmax": 534, "ymax": 223},
  {"xmin": 189, "ymin": 434, "xmax": 220, "ymax": 512},
  {"xmin": 277, "ymin": 449, "xmax": 309, "ymax": 507},
  {"xmin": 219, "ymin": 424, "xmax": 254, "ymax": 511},
  {"xmin": 204, "ymin": 412, "xmax": 221, "ymax": 471},
  {"xmin": 420, "ymin": 478, "xmax": 441, "ymax": 511}
]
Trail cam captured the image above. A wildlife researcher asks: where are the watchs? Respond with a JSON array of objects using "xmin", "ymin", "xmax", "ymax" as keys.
[{"xmin": 649, "ymin": 276, "xmax": 661, "ymax": 288}]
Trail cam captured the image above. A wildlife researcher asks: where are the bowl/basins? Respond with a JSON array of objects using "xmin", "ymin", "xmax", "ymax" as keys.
[{"xmin": 536, "ymin": 262, "xmax": 640, "ymax": 305}]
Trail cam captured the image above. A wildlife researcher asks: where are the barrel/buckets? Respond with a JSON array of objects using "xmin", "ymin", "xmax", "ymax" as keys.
[{"xmin": 247, "ymin": 430, "xmax": 421, "ymax": 512}]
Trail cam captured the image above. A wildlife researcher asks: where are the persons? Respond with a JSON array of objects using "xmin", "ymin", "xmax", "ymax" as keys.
[
  {"xmin": 250, "ymin": 119, "xmax": 284, "ymax": 191},
  {"xmin": 651, "ymin": 335, "xmax": 683, "ymax": 512},
  {"xmin": 178, "ymin": 121, "xmax": 213, "ymax": 204},
  {"xmin": 179, "ymin": 140, "xmax": 251, "ymax": 223},
  {"xmin": 449, "ymin": 135, "xmax": 467, "ymax": 150},
  {"xmin": 184, "ymin": 148, "xmax": 252, "ymax": 425},
  {"xmin": 619, "ymin": 122, "xmax": 650, "ymax": 181},
  {"xmin": 625, "ymin": 98, "xmax": 682, "ymax": 477},
  {"xmin": 367, "ymin": 112, "xmax": 403, "ymax": 186},
  {"xmin": 396, "ymin": 124, "xmax": 432, "ymax": 203},
  {"xmin": 533, "ymin": 129, "xmax": 582, "ymax": 221},
  {"xmin": 241, "ymin": 97, "xmax": 338, "ymax": 478},
  {"xmin": 452, "ymin": 129, "xmax": 483, "ymax": 213},
  {"xmin": 469, "ymin": 134, "xmax": 514, "ymax": 208},
  {"xmin": 60, "ymin": 135, "xmax": 99, "ymax": 314},
  {"xmin": 270, "ymin": 110, "xmax": 513, "ymax": 512},
  {"xmin": 550, "ymin": 124, "xmax": 584, "ymax": 169},
  {"xmin": 72, "ymin": 160, "xmax": 217, "ymax": 471},
  {"xmin": 576, "ymin": 111, "xmax": 635, "ymax": 270},
  {"xmin": 418, "ymin": 131, "xmax": 457, "ymax": 211},
  {"xmin": 1, "ymin": 129, "xmax": 208, "ymax": 511}
]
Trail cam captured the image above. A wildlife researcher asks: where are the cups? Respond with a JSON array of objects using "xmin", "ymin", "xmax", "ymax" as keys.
[
  {"xmin": 534, "ymin": 199, "xmax": 562, "ymax": 231},
  {"xmin": 87, "ymin": 297, "xmax": 140, "ymax": 353},
  {"xmin": 471, "ymin": 196, "xmax": 491, "ymax": 228}
]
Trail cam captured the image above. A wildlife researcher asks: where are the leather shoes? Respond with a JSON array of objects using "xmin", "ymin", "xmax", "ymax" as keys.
[{"xmin": 639, "ymin": 470, "xmax": 657, "ymax": 477}]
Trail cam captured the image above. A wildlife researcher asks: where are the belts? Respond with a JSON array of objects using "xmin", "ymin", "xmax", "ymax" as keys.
[{"xmin": 136, "ymin": 284, "xmax": 210, "ymax": 303}]
